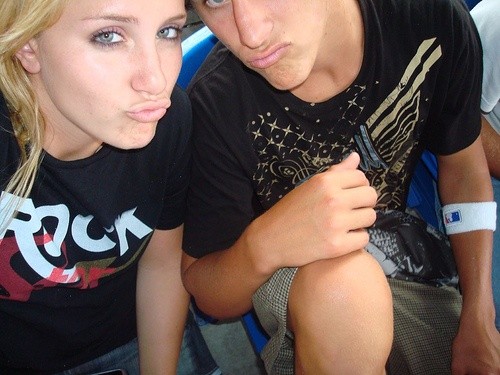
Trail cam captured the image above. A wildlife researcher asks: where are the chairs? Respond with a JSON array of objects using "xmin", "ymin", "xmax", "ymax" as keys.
[{"xmin": 177, "ymin": 26, "xmax": 440, "ymax": 375}]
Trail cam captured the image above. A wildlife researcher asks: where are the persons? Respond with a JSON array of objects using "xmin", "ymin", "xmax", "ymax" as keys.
[
  {"xmin": 0, "ymin": 0, "xmax": 190, "ymax": 375},
  {"xmin": 469, "ymin": 0, "xmax": 499, "ymax": 182},
  {"xmin": 181, "ymin": 0, "xmax": 499, "ymax": 375}
]
